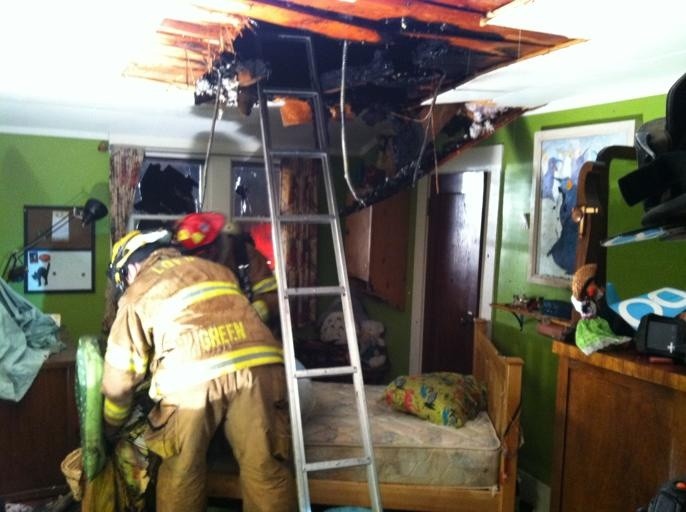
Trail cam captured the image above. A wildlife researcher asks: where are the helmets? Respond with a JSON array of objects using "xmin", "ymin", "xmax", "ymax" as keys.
[
  {"xmin": 106, "ymin": 227, "xmax": 170, "ymax": 289},
  {"xmin": 173, "ymin": 212, "xmax": 228, "ymax": 251}
]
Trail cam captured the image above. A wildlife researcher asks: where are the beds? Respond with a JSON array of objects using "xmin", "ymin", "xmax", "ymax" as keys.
[{"xmin": 75, "ymin": 317, "xmax": 524, "ymax": 510}]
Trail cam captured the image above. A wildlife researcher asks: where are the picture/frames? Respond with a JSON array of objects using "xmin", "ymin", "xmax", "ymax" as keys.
[{"xmin": 22, "ymin": 203, "xmax": 96, "ymax": 294}]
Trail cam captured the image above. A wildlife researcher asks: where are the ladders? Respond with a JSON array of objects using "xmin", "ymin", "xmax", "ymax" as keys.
[{"xmin": 257, "ymin": 28, "xmax": 382, "ymax": 512}]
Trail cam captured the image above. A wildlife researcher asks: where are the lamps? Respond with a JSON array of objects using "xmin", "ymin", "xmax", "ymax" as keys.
[{"xmin": 2, "ymin": 199, "xmax": 109, "ymax": 284}]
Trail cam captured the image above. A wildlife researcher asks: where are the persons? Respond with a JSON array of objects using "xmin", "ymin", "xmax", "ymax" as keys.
[
  {"xmin": 101, "ymin": 229, "xmax": 292, "ymax": 511},
  {"xmin": 174, "ymin": 213, "xmax": 279, "ymax": 324}
]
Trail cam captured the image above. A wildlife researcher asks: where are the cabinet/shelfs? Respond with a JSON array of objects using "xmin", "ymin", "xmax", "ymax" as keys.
[
  {"xmin": 546, "ymin": 339, "xmax": 685, "ymax": 511},
  {"xmin": 0, "ymin": 337, "xmax": 85, "ymax": 503}
]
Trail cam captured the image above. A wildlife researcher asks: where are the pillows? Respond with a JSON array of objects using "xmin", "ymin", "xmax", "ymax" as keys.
[{"xmin": 374, "ymin": 371, "xmax": 486, "ymax": 430}]
